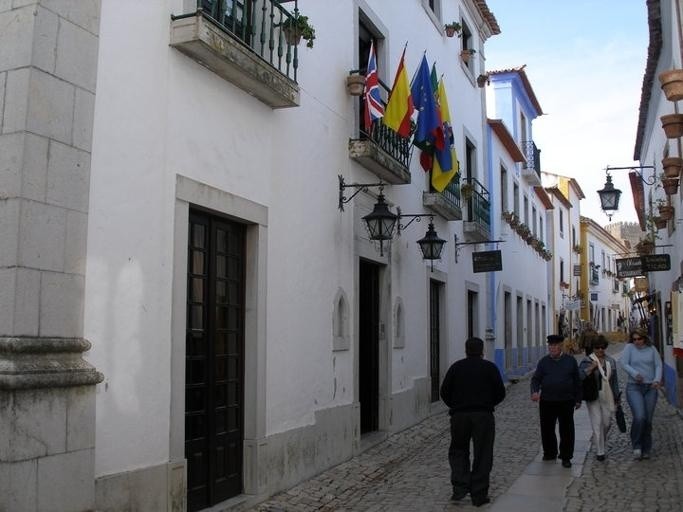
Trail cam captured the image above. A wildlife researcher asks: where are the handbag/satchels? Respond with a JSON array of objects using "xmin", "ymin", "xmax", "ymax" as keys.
[
  {"xmin": 581, "ymin": 373, "xmax": 598, "ymax": 401},
  {"xmin": 616, "ymin": 406, "xmax": 626, "ymax": 433}
]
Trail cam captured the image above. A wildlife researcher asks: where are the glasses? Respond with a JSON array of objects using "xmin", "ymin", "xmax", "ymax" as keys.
[
  {"xmin": 633, "ymin": 337, "xmax": 642, "ymax": 341},
  {"xmin": 594, "ymin": 344, "xmax": 606, "ymax": 349}
]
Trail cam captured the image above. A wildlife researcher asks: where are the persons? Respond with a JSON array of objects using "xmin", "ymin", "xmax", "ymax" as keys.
[
  {"xmin": 579, "ymin": 321, "xmax": 598, "ymax": 357},
  {"xmin": 576, "ymin": 334, "xmax": 622, "ymax": 461},
  {"xmin": 439, "ymin": 336, "xmax": 506, "ymax": 508},
  {"xmin": 620, "ymin": 324, "xmax": 663, "ymax": 459},
  {"xmin": 528, "ymin": 334, "xmax": 582, "ymax": 470}
]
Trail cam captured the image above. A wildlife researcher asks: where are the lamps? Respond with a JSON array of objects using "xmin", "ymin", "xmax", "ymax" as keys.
[
  {"xmin": 592, "ymin": 160, "xmax": 655, "ymax": 213},
  {"xmin": 335, "ymin": 171, "xmax": 400, "ymax": 260},
  {"xmin": 395, "ymin": 201, "xmax": 447, "ymax": 272},
  {"xmin": 644, "ymin": 300, "xmax": 657, "ymax": 315}
]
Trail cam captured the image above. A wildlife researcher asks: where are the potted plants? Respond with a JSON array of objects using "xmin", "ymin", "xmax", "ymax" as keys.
[
  {"xmin": 442, "ymin": 21, "xmax": 490, "ymax": 90},
  {"xmin": 282, "ymin": 13, "xmax": 315, "ymax": 51},
  {"xmin": 503, "ymin": 212, "xmax": 554, "ymax": 263}
]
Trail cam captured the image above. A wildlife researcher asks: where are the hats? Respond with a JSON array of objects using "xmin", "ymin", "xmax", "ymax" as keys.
[{"xmin": 547, "ymin": 334, "xmax": 564, "ymax": 343}]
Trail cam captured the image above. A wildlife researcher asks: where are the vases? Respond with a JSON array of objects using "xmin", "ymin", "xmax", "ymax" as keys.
[
  {"xmin": 343, "ymin": 73, "xmax": 367, "ymax": 97},
  {"xmin": 635, "ymin": 68, "xmax": 683, "ymax": 254}
]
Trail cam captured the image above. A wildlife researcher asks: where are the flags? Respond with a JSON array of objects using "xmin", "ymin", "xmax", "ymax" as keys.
[{"xmin": 361, "ymin": 40, "xmax": 460, "ymax": 193}]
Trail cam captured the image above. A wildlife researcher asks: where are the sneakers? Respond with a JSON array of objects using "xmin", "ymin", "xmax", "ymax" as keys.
[
  {"xmin": 560, "ymin": 458, "xmax": 571, "ymax": 467},
  {"xmin": 634, "ymin": 449, "xmax": 642, "ymax": 459}
]
